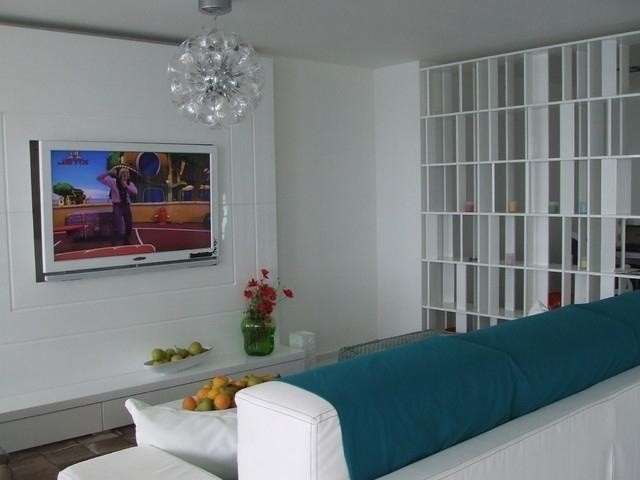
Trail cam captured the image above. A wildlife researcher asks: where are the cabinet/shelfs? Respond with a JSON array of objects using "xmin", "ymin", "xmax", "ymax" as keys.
[
  {"xmin": 0, "ymin": 346, "xmax": 308, "ymax": 456},
  {"xmin": 418, "ymin": 29, "xmax": 639, "ymax": 333}
]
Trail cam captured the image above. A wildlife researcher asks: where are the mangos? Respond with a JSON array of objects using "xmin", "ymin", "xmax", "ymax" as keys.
[{"xmin": 194, "ymin": 376, "xmax": 248, "ymax": 408}]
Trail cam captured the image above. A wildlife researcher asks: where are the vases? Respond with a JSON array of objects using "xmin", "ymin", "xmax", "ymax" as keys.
[{"xmin": 241, "ymin": 315, "xmax": 275, "ymax": 356}]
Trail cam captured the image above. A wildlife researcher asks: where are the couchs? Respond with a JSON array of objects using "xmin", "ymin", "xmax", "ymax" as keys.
[{"xmin": 57, "ymin": 288, "xmax": 640, "ymax": 480}]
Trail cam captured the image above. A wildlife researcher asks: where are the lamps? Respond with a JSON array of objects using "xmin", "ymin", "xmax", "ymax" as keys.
[{"xmin": 165, "ymin": 1, "xmax": 265, "ymax": 131}]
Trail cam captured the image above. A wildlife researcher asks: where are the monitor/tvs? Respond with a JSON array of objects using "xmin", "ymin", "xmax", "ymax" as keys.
[{"xmin": 29, "ymin": 139, "xmax": 220, "ymax": 282}]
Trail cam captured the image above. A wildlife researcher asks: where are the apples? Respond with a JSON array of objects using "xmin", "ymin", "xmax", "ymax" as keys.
[
  {"xmin": 183, "ymin": 396, "xmax": 196, "ymax": 410},
  {"xmin": 151, "ymin": 342, "xmax": 209, "ymax": 366},
  {"xmin": 247, "ymin": 373, "xmax": 263, "ymax": 387},
  {"xmin": 198, "ymin": 395, "xmax": 231, "ymax": 411}
]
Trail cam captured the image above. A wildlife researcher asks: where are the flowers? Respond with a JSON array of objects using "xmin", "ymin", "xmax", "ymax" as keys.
[{"xmin": 243, "ymin": 267, "xmax": 293, "ymax": 346}]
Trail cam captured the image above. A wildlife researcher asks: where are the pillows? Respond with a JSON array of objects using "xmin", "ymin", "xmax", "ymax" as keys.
[{"xmin": 124, "ymin": 398, "xmax": 238, "ymax": 480}]
